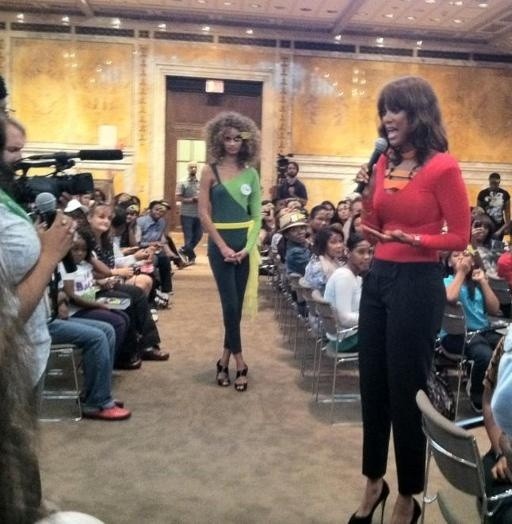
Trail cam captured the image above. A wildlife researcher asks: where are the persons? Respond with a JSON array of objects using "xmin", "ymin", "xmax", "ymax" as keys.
[
  {"xmin": 274, "ymin": 162, "xmax": 308, "ymax": 203},
  {"xmin": 441, "ymin": 172, "xmax": 512, "ymax": 479},
  {"xmin": 175, "ymin": 162, "xmax": 203, "ymax": 264},
  {"xmin": 198, "ymin": 113, "xmax": 262, "ymax": 391},
  {"xmin": 259, "ymin": 198, "xmax": 370, "ymax": 350},
  {"xmin": 45, "ymin": 189, "xmax": 195, "ymax": 420},
  {"xmin": 0, "ymin": 77, "xmax": 97, "ymax": 523},
  {"xmin": 348, "ymin": 77, "xmax": 471, "ymax": 523}
]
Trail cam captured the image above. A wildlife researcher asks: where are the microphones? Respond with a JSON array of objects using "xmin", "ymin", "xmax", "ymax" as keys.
[
  {"xmin": 355, "ymin": 138, "xmax": 388, "ymax": 192},
  {"xmin": 35, "ymin": 192, "xmax": 78, "ymax": 274}
]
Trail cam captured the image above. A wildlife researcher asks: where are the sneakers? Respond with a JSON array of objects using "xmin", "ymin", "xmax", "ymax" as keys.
[
  {"xmin": 125, "ymin": 351, "xmax": 141, "ymax": 368},
  {"xmin": 76, "ymin": 394, "xmax": 124, "ymax": 406},
  {"xmin": 141, "ymin": 345, "xmax": 169, "ymax": 360},
  {"xmin": 82, "ymin": 407, "xmax": 130, "ymax": 419}
]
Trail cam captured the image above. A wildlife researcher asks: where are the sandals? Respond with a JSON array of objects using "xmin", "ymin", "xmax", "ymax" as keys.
[
  {"xmin": 234, "ymin": 365, "xmax": 248, "ymax": 391},
  {"xmin": 217, "ymin": 359, "xmax": 230, "ymax": 386}
]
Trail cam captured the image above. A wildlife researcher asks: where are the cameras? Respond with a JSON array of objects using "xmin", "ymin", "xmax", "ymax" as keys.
[{"xmin": 132, "ymin": 264, "xmax": 141, "ymax": 275}]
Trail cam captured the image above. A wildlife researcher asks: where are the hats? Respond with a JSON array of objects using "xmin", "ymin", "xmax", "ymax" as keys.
[
  {"xmin": 64, "ymin": 198, "xmax": 90, "ymax": 213},
  {"xmin": 277, "ymin": 212, "xmax": 309, "ymax": 232}
]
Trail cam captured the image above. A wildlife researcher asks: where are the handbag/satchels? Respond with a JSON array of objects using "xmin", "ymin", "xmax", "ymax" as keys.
[{"xmin": 426, "ymin": 367, "xmax": 455, "ymax": 419}]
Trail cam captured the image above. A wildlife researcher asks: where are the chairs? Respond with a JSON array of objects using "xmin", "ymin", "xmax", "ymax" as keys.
[
  {"xmin": 429, "ymin": 233, "xmax": 512, "ymax": 428},
  {"xmin": 35, "ymin": 344, "xmax": 84, "ymax": 423},
  {"xmin": 416, "ymin": 389, "xmax": 512, "ymax": 524},
  {"xmin": 259, "ymin": 233, "xmax": 358, "ymax": 426}
]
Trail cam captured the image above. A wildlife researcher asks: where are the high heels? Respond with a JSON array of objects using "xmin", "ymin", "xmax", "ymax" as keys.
[
  {"xmin": 347, "ymin": 478, "xmax": 389, "ymax": 524},
  {"xmin": 410, "ymin": 497, "xmax": 422, "ymax": 524}
]
